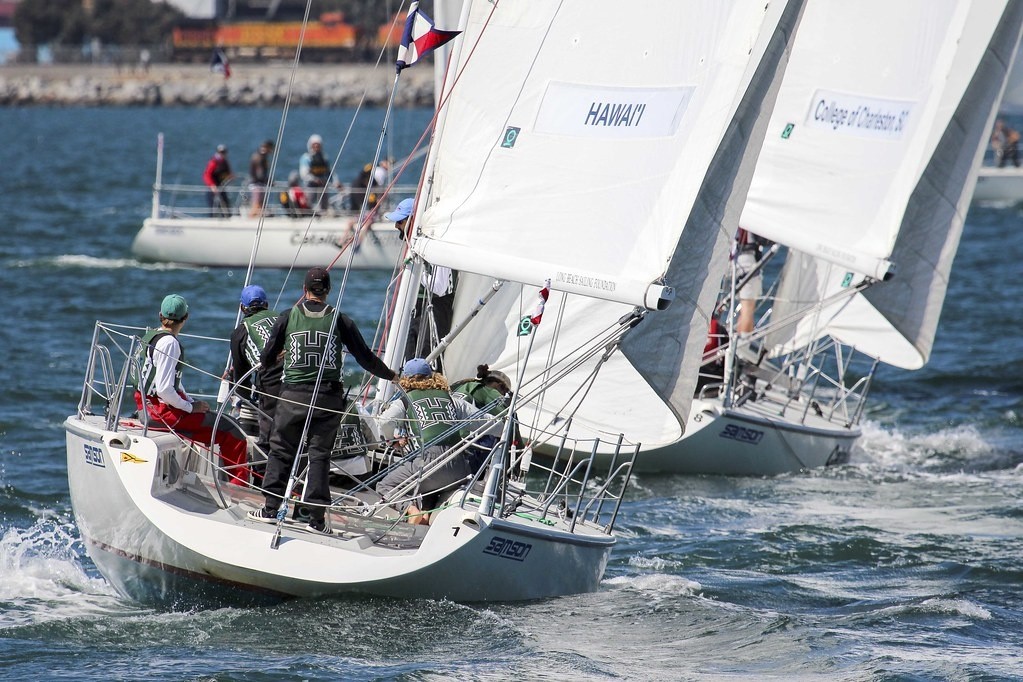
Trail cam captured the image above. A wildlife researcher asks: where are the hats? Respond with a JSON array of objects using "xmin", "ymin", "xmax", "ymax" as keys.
[
  {"xmin": 375, "ymin": 167, "xmax": 386, "ymax": 186},
  {"xmin": 386, "ymin": 198, "xmax": 417, "ymax": 220},
  {"xmin": 487, "ymin": 370, "xmax": 511, "ymax": 391},
  {"xmin": 241, "ymin": 285, "xmax": 268, "ymax": 308},
  {"xmin": 304, "ymin": 268, "xmax": 331, "ymax": 295},
  {"xmin": 160, "ymin": 294, "xmax": 188, "ymax": 321},
  {"xmin": 403, "ymin": 358, "xmax": 433, "ymax": 377}
]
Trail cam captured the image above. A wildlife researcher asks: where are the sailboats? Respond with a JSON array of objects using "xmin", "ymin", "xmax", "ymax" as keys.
[{"xmin": 62, "ymin": 0, "xmax": 1023, "ymax": 616}]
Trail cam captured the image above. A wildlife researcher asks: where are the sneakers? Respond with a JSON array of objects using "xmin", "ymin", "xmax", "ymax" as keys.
[
  {"xmin": 247, "ymin": 509, "xmax": 278, "ymax": 523},
  {"xmin": 306, "ymin": 523, "xmax": 336, "ymax": 536}
]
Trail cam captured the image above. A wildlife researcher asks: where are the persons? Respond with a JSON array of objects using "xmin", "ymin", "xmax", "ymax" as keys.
[
  {"xmin": 230, "ymin": 284, "xmax": 284, "ymax": 491},
  {"xmin": 247, "ymin": 266, "xmax": 398, "ymax": 536},
  {"xmin": 385, "ymin": 199, "xmax": 458, "ymax": 363},
  {"xmin": 727, "ymin": 229, "xmax": 763, "ymax": 333},
  {"xmin": 203, "ymin": 143, "xmax": 233, "ymax": 217},
  {"xmin": 449, "ymin": 364, "xmax": 526, "ymax": 480},
  {"xmin": 379, "ymin": 358, "xmax": 505, "ymax": 526},
  {"xmin": 694, "ymin": 318, "xmax": 730, "ymax": 394},
  {"xmin": 992, "ymin": 119, "xmax": 1021, "ymax": 168},
  {"xmin": 351, "ymin": 157, "xmax": 396, "ymax": 210},
  {"xmin": 279, "ymin": 171, "xmax": 308, "ymax": 218},
  {"xmin": 299, "ymin": 134, "xmax": 342, "ymax": 209},
  {"xmin": 132, "ymin": 293, "xmax": 249, "ymax": 487},
  {"xmin": 248, "ymin": 141, "xmax": 274, "ymax": 218}
]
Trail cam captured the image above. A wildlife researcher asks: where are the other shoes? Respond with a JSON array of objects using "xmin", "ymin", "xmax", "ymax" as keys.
[{"xmin": 408, "ymin": 514, "xmax": 424, "ymax": 525}]
